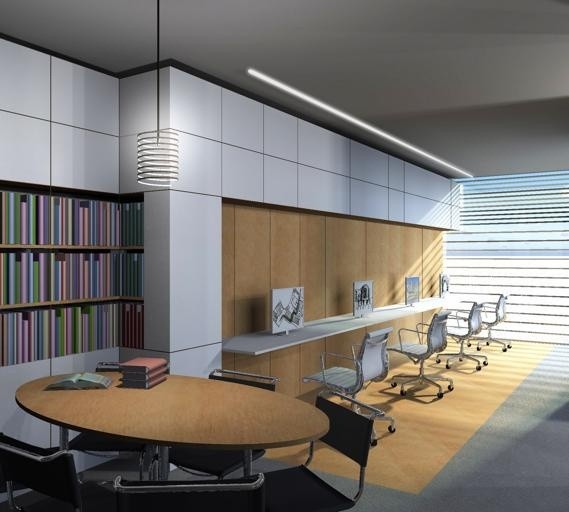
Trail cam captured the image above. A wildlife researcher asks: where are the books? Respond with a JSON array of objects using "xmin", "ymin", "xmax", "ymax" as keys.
[
  {"xmin": 118, "ymin": 375, "xmax": 167, "ymax": 389},
  {"xmin": 119, "ymin": 355, "xmax": 168, "ymax": 373},
  {"xmin": 42, "ymin": 371, "xmax": 112, "ymax": 389},
  {"xmin": 0, "ymin": 192, "xmax": 144, "ymax": 364},
  {"xmin": 120, "ymin": 365, "xmax": 170, "ymax": 382}
]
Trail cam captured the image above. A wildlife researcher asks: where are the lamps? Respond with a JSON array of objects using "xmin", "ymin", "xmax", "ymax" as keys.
[{"xmin": 136, "ymin": 0, "xmax": 181, "ymax": 189}]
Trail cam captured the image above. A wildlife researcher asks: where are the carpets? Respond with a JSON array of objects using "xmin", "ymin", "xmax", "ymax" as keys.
[{"xmin": 262, "ymin": 337, "xmax": 554, "ymax": 497}]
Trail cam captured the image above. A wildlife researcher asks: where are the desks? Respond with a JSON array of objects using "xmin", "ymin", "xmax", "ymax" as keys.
[{"xmin": 14, "ymin": 372, "xmax": 330, "ymax": 479}]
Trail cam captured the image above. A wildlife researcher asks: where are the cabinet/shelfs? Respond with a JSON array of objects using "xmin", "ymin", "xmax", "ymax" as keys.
[{"xmin": 0, "ymin": 188, "xmax": 147, "ymax": 368}]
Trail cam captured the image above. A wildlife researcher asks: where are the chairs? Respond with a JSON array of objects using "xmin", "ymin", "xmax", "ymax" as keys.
[
  {"xmin": 0, "ymin": 431, "xmax": 83, "ymax": 512},
  {"xmin": 296, "ymin": 292, "xmax": 515, "ymax": 448},
  {"xmin": 68, "ymin": 360, "xmax": 386, "ymax": 512}
]
